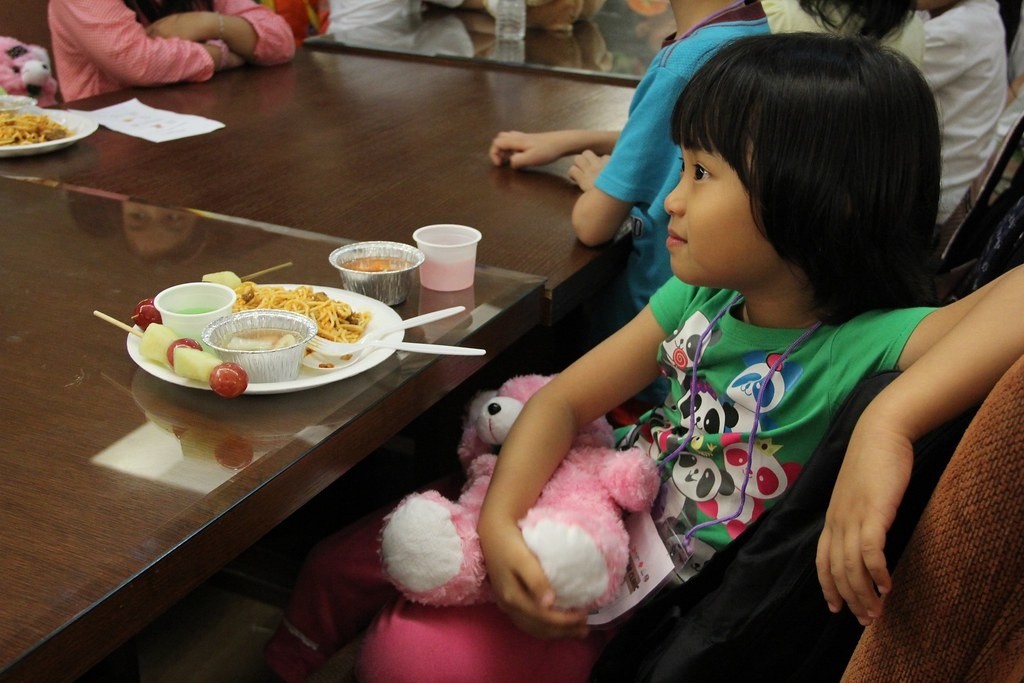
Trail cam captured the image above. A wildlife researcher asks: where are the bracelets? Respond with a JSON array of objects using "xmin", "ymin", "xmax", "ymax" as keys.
[{"xmin": 214, "ymin": 10, "xmax": 224, "ymax": 41}]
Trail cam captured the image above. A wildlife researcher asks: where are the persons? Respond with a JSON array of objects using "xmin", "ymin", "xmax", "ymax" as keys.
[
  {"xmin": 760, "ymin": 0, "xmax": 924, "ymax": 73},
  {"xmin": 297, "ymin": 0, "xmax": 773, "ymax": 528},
  {"xmin": 120, "ymin": 201, "xmax": 211, "ymax": 270},
  {"xmin": 910, "ymin": 0, "xmax": 1009, "ymax": 230},
  {"xmin": 49, "ymin": 1, "xmax": 294, "ymax": 104},
  {"xmin": 261, "ymin": 34, "xmax": 1022, "ymax": 683}
]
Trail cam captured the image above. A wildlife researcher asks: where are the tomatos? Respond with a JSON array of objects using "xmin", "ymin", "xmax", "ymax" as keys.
[
  {"xmin": 213, "ymin": 435, "xmax": 254, "ymax": 470},
  {"xmin": 167, "ymin": 337, "xmax": 203, "ymax": 369},
  {"xmin": 132, "ymin": 297, "xmax": 163, "ymax": 330},
  {"xmin": 171, "ymin": 424, "xmax": 188, "ymax": 439},
  {"xmin": 210, "ymin": 362, "xmax": 249, "ymax": 400}
]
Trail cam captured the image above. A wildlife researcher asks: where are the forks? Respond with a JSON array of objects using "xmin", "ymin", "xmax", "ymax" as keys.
[{"xmin": 306, "ymin": 335, "xmax": 486, "ymax": 356}]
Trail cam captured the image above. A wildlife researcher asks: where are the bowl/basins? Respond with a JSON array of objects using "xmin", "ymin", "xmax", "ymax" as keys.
[
  {"xmin": 0, "ymin": 95, "xmax": 39, "ymax": 118},
  {"xmin": 329, "ymin": 241, "xmax": 426, "ymax": 305},
  {"xmin": 202, "ymin": 308, "xmax": 318, "ymax": 384}
]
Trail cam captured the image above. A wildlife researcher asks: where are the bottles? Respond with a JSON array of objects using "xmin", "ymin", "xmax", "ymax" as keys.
[{"xmin": 495, "ymin": 0, "xmax": 527, "ymax": 41}]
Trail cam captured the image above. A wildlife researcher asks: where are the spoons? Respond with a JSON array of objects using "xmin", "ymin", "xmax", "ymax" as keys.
[{"xmin": 301, "ymin": 306, "xmax": 465, "ymax": 371}]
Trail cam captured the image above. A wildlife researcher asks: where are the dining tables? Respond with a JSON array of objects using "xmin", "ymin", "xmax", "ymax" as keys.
[
  {"xmin": 302, "ymin": 0, "xmax": 677, "ymax": 85},
  {"xmin": 1, "ymin": 175, "xmax": 553, "ymax": 683},
  {"xmin": 0, "ymin": 45, "xmax": 645, "ymax": 310}
]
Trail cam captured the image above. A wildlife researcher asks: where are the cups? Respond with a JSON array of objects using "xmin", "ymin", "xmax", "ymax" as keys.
[
  {"xmin": 153, "ymin": 283, "xmax": 236, "ymax": 352},
  {"xmin": 412, "ymin": 223, "xmax": 482, "ymax": 292}
]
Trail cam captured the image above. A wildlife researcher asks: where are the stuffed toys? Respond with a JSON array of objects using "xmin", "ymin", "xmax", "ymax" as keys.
[
  {"xmin": 377, "ymin": 370, "xmax": 659, "ymax": 614},
  {"xmin": 1, "ymin": 36, "xmax": 58, "ymax": 108}
]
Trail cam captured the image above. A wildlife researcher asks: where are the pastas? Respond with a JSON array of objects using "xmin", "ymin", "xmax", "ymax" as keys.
[
  {"xmin": 0, "ymin": 112, "xmax": 69, "ymax": 147},
  {"xmin": 232, "ymin": 280, "xmax": 370, "ymax": 345}
]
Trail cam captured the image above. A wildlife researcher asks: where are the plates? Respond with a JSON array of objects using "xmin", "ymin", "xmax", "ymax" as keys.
[
  {"xmin": 126, "ymin": 282, "xmax": 406, "ymax": 395},
  {"xmin": 0, "ymin": 109, "xmax": 99, "ymax": 159}
]
